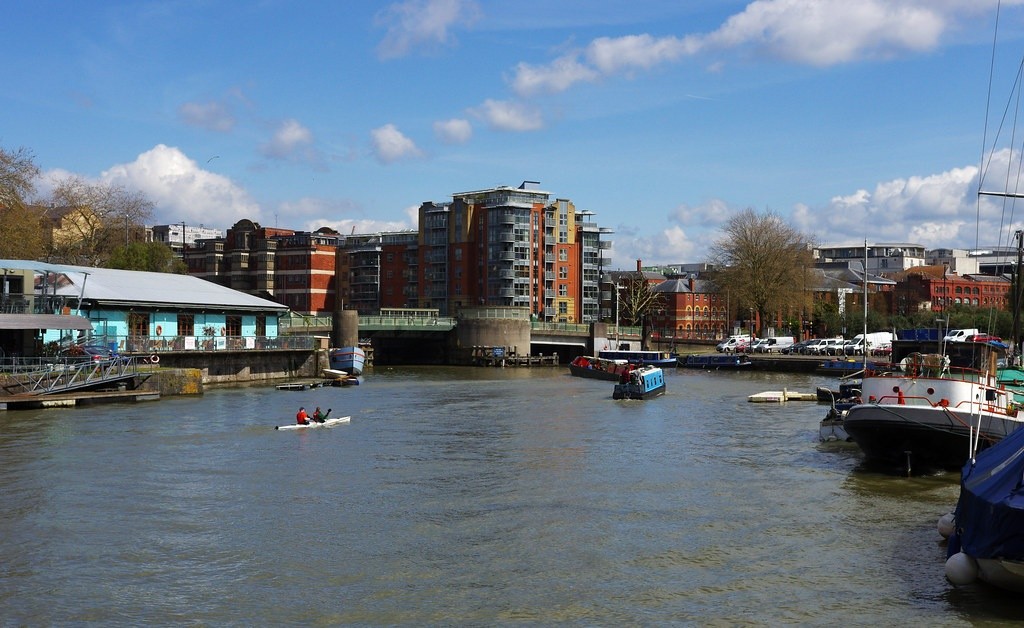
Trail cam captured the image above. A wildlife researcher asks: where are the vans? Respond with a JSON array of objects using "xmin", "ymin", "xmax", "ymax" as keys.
[
  {"xmin": 843, "ymin": 332, "xmax": 898, "ymax": 356},
  {"xmin": 942, "ymin": 328, "xmax": 979, "ymax": 342},
  {"xmin": 756, "ymin": 336, "xmax": 793, "ymax": 353}
]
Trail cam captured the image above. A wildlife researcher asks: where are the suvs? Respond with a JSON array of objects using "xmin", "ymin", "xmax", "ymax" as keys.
[{"xmin": 964, "ymin": 333, "xmax": 1002, "ymax": 344}]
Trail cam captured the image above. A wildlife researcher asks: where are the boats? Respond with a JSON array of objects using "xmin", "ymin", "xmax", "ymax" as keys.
[
  {"xmin": 935, "ymin": 422, "xmax": 1024, "ymax": 589},
  {"xmin": 611, "ymin": 364, "xmax": 667, "ymax": 400},
  {"xmin": 345, "ymin": 376, "xmax": 364, "ymax": 386},
  {"xmin": 274, "ymin": 415, "xmax": 351, "ymax": 431},
  {"xmin": 598, "ymin": 348, "xmax": 679, "ymax": 369},
  {"xmin": 320, "ymin": 369, "xmax": 348, "ymax": 377},
  {"xmin": 329, "ymin": 347, "xmax": 364, "ymax": 375},
  {"xmin": 568, "ymin": 354, "xmax": 638, "ymax": 381},
  {"xmin": 835, "ymin": 330, "xmax": 1024, "ymax": 473}
]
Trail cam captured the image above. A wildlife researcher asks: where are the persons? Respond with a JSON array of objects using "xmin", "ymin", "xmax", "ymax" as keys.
[
  {"xmin": 622, "ymin": 366, "xmax": 631, "ymax": 385},
  {"xmin": 296, "ymin": 407, "xmax": 313, "ymax": 425},
  {"xmin": 633, "ymin": 365, "xmax": 644, "ymax": 385},
  {"xmin": 313, "ymin": 407, "xmax": 325, "ymax": 423}
]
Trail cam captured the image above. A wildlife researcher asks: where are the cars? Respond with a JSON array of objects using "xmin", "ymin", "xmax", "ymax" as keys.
[
  {"xmin": 778, "ymin": 337, "xmax": 853, "ymax": 356},
  {"xmin": 869, "ymin": 341, "xmax": 893, "ymax": 357},
  {"xmin": 717, "ymin": 334, "xmax": 769, "ymax": 352}
]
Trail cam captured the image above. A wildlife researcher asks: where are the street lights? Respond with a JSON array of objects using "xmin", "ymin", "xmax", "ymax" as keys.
[
  {"xmin": 657, "ymin": 309, "xmax": 662, "ymax": 351},
  {"xmin": 841, "ymin": 312, "xmax": 845, "ymax": 356},
  {"xmin": 748, "ymin": 306, "xmax": 753, "ymax": 354}
]
